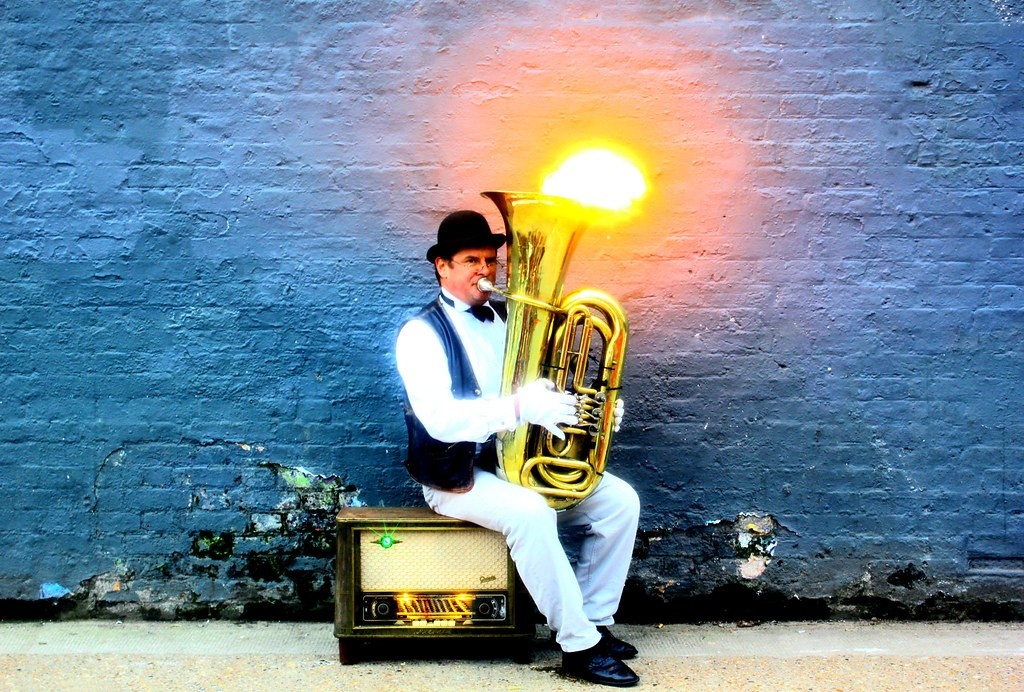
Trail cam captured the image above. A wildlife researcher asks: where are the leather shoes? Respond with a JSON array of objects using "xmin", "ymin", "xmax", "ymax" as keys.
[
  {"xmin": 561, "ymin": 643, "xmax": 639, "ymax": 687},
  {"xmin": 550, "ymin": 626, "xmax": 639, "ymax": 657}
]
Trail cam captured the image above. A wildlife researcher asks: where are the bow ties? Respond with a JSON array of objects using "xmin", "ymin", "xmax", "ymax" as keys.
[{"xmin": 440, "ymin": 290, "xmax": 494, "ymax": 323}]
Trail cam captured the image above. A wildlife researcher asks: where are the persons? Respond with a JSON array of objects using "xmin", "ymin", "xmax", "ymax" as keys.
[{"xmin": 395, "ymin": 210, "xmax": 640, "ymax": 687}]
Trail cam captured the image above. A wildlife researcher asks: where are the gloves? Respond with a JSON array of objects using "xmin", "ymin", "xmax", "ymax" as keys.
[
  {"xmin": 517, "ymin": 379, "xmax": 578, "ymax": 440},
  {"xmin": 614, "ymin": 399, "xmax": 624, "ymax": 432}
]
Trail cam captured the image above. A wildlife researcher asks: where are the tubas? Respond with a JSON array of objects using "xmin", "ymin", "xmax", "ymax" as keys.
[{"xmin": 477, "ymin": 189, "xmax": 628, "ymax": 511}]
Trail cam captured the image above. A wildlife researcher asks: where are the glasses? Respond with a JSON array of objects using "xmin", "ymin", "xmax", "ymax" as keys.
[{"xmin": 452, "ymin": 258, "xmax": 503, "ymax": 272}]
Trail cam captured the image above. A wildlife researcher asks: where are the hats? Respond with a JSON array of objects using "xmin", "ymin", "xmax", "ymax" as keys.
[{"xmin": 427, "ymin": 210, "xmax": 507, "ymax": 264}]
